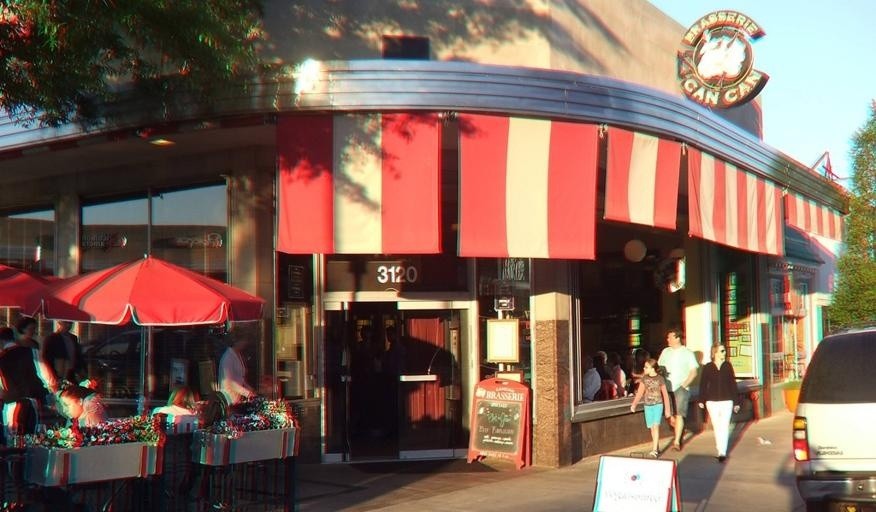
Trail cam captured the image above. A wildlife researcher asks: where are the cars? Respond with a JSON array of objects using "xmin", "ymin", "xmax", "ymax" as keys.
[{"xmin": 82, "ymin": 328, "xmax": 223, "ymax": 389}]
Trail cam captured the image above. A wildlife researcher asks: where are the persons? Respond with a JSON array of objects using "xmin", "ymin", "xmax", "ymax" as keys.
[
  {"xmin": 216, "ymin": 326, "xmax": 258, "ymax": 408},
  {"xmin": 697, "ymin": 341, "xmax": 741, "ymax": 465},
  {"xmin": 0, "ymin": 317, "xmax": 89, "ymax": 482},
  {"xmin": 656, "ymin": 327, "xmax": 699, "ymax": 452},
  {"xmin": 629, "ymin": 358, "xmax": 671, "ymax": 459},
  {"xmin": 355, "ymin": 324, "xmax": 396, "ymax": 372},
  {"xmin": 165, "ymin": 356, "xmax": 202, "ymax": 408},
  {"xmin": 580, "ymin": 344, "xmax": 650, "ymax": 403}
]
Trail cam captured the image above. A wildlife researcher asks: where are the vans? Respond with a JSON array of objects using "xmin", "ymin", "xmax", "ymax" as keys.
[{"xmin": 793, "ymin": 327, "xmax": 876, "ymax": 512}]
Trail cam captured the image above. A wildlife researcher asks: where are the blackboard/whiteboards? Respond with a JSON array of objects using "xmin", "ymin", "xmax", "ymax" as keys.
[
  {"xmin": 589, "ymin": 455, "xmax": 677, "ymax": 512},
  {"xmin": 473, "ymin": 398, "xmax": 523, "ymax": 456}
]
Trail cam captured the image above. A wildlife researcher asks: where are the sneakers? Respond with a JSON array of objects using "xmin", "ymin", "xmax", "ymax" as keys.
[
  {"xmin": 719, "ymin": 456, "xmax": 727, "ymax": 462},
  {"xmin": 647, "ymin": 443, "xmax": 682, "ymax": 459}
]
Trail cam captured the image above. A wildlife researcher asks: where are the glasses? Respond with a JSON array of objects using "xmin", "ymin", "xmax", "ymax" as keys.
[{"xmin": 721, "ymin": 350, "xmax": 727, "ymax": 353}]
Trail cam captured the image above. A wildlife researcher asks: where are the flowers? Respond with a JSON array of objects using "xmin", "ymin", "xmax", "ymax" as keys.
[
  {"xmin": 38, "ymin": 413, "xmax": 164, "ymax": 447},
  {"xmin": 205, "ymin": 398, "xmax": 293, "ymax": 439}
]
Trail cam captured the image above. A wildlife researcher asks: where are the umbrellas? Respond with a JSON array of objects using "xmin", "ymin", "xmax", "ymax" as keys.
[
  {"xmin": 17, "ymin": 251, "xmax": 267, "ymax": 414},
  {"xmin": 0, "ymin": 263, "xmax": 60, "ymax": 327}
]
